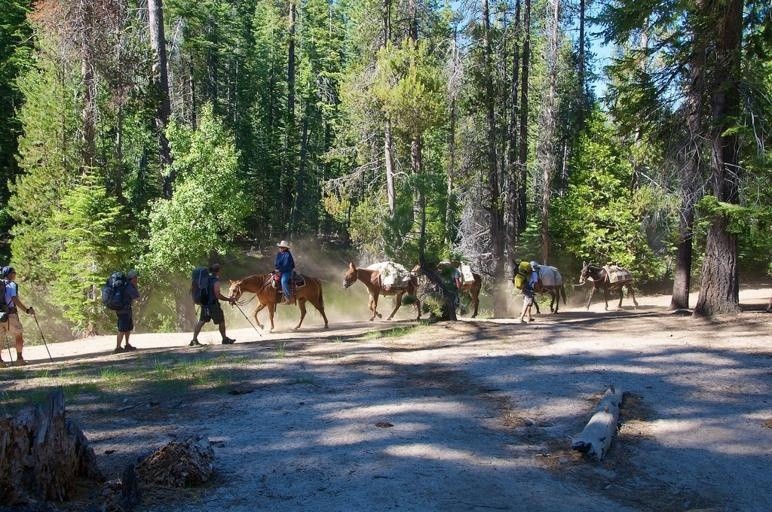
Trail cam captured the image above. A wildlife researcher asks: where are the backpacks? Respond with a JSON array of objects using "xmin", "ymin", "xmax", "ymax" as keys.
[
  {"xmin": 515, "ymin": 261, "xmax": 532, "ymax": 289},
  {"xmin": 192, "ymin": 268, "xmax": 218, "ymax": 306},
  {"xmin": 0, "ymin": 280, "xmax": 18, "ymax": 313},
  {"xmin": 102, "ymin": 271, "xmax": 134, "ymax": 310}
]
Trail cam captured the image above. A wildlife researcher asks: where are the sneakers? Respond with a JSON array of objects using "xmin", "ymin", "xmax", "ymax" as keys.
[
  {"xmin": 222, "ymin": 338, "xmax": 234, "ymax": 344},
  {"xmin": 0, "ymin": 362, "xmax": 6, "ymax": 368},
  {"xmin": 125, "ymin": 345, "xmax": 135, "ymax": 351},
  {"xmin": 16, "ymin": 358, "xmax": 26, "ymax": 364},
  {"xmin": 115, "ymin": 347, "xmax": 123, "ymax": 352},
  {"xmin": 281, "ymin": 300, "xmax": 290, "ymax": 305},
  {"xmin": 190, "ymin": 341, "xmax": 200, "ymax": 345}
]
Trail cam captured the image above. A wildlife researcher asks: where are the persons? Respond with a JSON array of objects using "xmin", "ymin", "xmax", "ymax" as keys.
[
  {"xmin": 0, "ymin": 266, "xmax": 35, "ymax": 368},
  {"xmin": 437, "ymin": 259, "xmax": 462, "ymax": 320},
  {"xmin": 114, "ymin": 271, "xmax": 140, "ymax": 353},
  {"xmin": 188, "ymin": 263, "xmax": 236, "ymax": 348},
  {"xmin": 520, "ymin": 260, "xmax": 539, "ymax": 323},
  {"xmin": 275, "ymin": 239, "xmax": 295, "ymax": 305}
]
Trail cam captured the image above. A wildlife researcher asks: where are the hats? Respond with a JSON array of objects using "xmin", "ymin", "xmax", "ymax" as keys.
[
  {"xmin": 127, "ymin": 272, "xmax": 137, "ymax": 280},
  {"xmin": 3, "ymin": 267, "xmax": 11, "ymax": 276},
  {"xmin": 276, "ymin": 240, "xmax": 290, "ymax": 248},
  {"xmin": 210, "ymin": 264, "xmax": 219, "ymax": 272}
]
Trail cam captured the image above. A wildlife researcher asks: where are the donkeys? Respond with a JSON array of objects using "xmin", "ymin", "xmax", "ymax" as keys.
[
  {"xmin": 410, "ymin": 254, "xmax": 482, "ymax": 318},
  {"xmin": 342, "ymin": 261, "xmax": 422, "ymax": 321},
  {"xmin": 579, "ymin": 260, "xmax": 638, "ymax": 312},
  {"xmin": 511, "ymin": 258, "xmax": 567, "ymax": 314}
]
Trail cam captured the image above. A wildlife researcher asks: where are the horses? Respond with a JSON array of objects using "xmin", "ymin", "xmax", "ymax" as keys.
[{"xmin": 228, "ymin": 274, "xmax": 330, "ymax": 333}]
